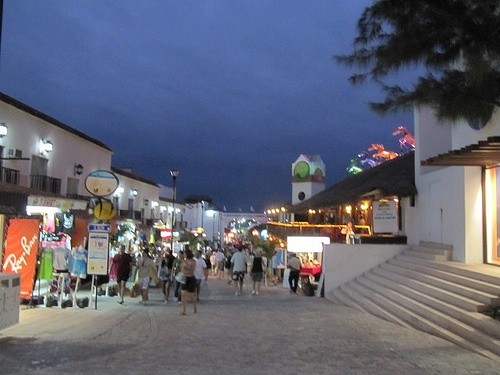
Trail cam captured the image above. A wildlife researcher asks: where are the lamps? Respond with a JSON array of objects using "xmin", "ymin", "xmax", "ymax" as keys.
[
  {"xmin": 133, "ymin": 189, "xmax": 138, "ymax": 196},
  {"xmin": 39, "ymin": 139, "xmax": 53, "ymax": 156},
  {"xmin": 74, "ymin": 164, "xmax": 83, "ymax": 177},
  {"xmin": 0, "ymin": 123, "xmax": 7, "ymax": 138},
  {"xmin": 144, "ymin": 199, "xmax": 149, "ymax": 206}
]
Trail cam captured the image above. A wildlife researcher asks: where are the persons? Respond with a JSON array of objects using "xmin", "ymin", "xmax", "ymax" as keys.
[
  {"xmin": 35, "ymin": 243, "xmax": 88, "ymax": 281},
  {"xmin": 249, "ymin": 249, "xmax": 269, "ymax": 296},
  {"xmin": 109, "ymin": 241, "xmax": 252, "ymax": 287},
  {"xmin": 229, "ymin": 244, "xmax": 250, "ymax": 296},
  {"xmin": 156, "ymin": 247, "xmax": 176, "ymax": 304},
  {"xmin": 108, "ymin": 245, "xmax": 135, "ymax": 304},
  {"xmin": 271, "ymin": 250, "xmax": 282, "ymax": 283},
  {"xmin": 171, "ymin": 251, "xmax": 182, "ymax": 304},
  {"xmin": 325, "ymin": 216, "xmax": 369, "ymax": 246},
  {"xmin": 287, "ymin": 252, "xmax": 304, "ymax": 295},
  {"xmin": 180, "ymin": 249, "xmax": 198, "ymax": 315},
  {"xmin": 193, "ymin": 251, "xmax": 208, "ymax": 302},
  {"xmin": 133, "ymin": 248, "xmax": 158, "ymax": 304}
]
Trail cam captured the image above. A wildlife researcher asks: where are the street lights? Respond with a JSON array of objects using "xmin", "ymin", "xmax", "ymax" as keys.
[{"xmin": 169, "ymin": 170, "xmax": 179, "ymax": 252}]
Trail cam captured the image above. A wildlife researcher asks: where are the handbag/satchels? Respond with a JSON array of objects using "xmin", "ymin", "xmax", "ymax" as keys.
[
  {"xmin": 131, "ymin": 283, "xmax": 140, "ymax": 298},
  {"xmin": 176, "ymin": 271, "xmax": 187, "ymax": 285}
]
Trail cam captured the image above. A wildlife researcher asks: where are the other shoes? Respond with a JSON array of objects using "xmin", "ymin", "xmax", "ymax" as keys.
[
  {"xmin": 252, "ymin": 290, "xmax": 255, "ymax": 295},
  {"xmin": 164, "ymin": 300, "xmax": 168, "ymax": 304},
  {"xmin": 256, "ymin": 292, "xmax": 259, "ymax": 296},
  {"xmin": 118, "ymin": 298, "xmax": 123, "ymax": 304}
]
